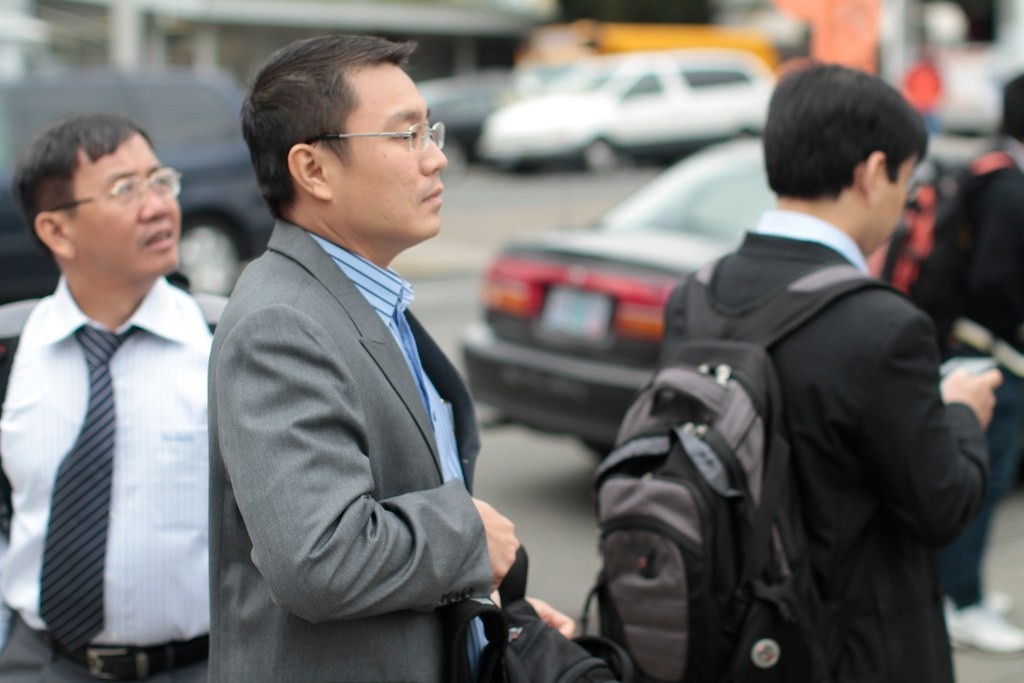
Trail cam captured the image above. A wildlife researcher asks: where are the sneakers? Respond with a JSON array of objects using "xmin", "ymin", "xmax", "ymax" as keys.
[{"xmin": 945, "ymin": 597, "xmax": 1024, "ymax": 659}]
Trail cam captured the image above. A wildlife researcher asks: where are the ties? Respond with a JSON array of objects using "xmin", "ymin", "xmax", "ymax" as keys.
[{"xmin": 37, "ymin": 329, "xmax": 137, "ymax": 653}]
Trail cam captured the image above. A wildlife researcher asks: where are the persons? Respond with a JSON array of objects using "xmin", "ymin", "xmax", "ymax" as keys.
[
  {"xmin": 915, "ymin": 73, "xmax": 1024, "ymax": 652},
  {"xmin": 0, "ymin": 113, "xmax": 214, "ymax": 683},
  {"xmin": 208, "ymin": 35, "xmax": 574, "ymax": 683},
  {"xmin": 662, "ymin": 62, "xmax": 1002, "ymax": 683}
]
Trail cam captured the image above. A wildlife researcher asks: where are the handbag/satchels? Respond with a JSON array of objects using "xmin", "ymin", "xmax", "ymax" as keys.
[{"xmin": 438, "ymin": 544, "xmax": 615, "ymax": 683}]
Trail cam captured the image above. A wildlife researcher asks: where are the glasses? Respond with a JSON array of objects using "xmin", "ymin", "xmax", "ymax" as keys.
[
  {"xmin": 46, "ymin": 168, "xmax": 183, "ymax": 210},
  {"xmin": 307, "ymin": 123, "xmax": 446, "ymax": 153}
]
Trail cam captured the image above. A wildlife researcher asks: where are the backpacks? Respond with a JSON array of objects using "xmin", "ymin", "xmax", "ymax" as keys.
[
  {"xmin": 866, "ymin": 151, "xmax": 1011, "ymax": 315},
  {"xmin": 590, "ymin": 265, "xmax": 887, "ymax": 683}
]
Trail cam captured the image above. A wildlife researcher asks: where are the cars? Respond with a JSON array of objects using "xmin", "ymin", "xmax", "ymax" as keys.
[
  {"xmin": 476, "ymin": 47, "xmax": 777, "ymax": 173},
  {"xmin": 0, "ymin": 68, "xmax": 279, "ymax": 305},
  {"xmin": 458, "ymin": 137, "xmax": 778, "ymax": 461}
]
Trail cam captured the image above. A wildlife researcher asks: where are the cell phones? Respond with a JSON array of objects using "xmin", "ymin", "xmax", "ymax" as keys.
[{"xmin": 940, "ymin": 356, "xmax": 998, "ymax": 380}]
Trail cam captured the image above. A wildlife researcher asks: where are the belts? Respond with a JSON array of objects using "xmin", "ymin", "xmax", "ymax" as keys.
[{"xmin": 37, "ymin": 634, "xmax": 209, "ymax": 683}]
